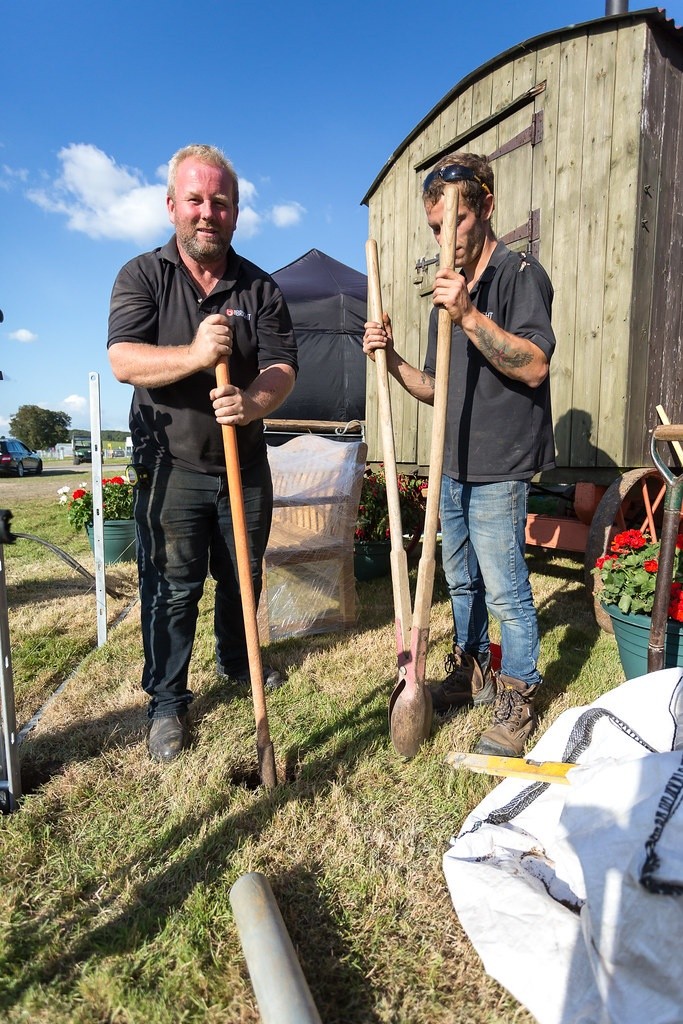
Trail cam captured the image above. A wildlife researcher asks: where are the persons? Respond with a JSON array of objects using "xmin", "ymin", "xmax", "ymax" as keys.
[
  {"xmin": 362, "ymin": 153, "xmax": 558, "ymax": 759},
  {"xmin": 107, "ymin": 142, "xmax": 300, "ymax": 765}
]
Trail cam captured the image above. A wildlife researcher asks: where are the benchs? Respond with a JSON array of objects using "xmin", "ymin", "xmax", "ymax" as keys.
[{"xmin": 258, "ymin": 435, "xmax": 369, "ymax": 649}]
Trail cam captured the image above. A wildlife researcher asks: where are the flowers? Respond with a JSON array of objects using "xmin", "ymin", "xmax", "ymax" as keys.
[
  {"xmin": 590, "ymin": 528, "xmax": 683, "ymax": 624},
  {"xmin": 352, "ymin": 467, "xmax": 430, "ymax": 543},
  {"xmin": 57, "ymin": 475, "xmax": 135, "ymax": 535}
]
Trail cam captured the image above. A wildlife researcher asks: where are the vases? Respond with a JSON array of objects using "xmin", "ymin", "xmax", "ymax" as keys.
[
  {"xmin": 354, "ymin": 541, "xmax": 391, "ymax": 583},
  {"xmin": 592, "ymin": 572, "xmax": 617, "ymax": 634},
  {"xmin": 84, "ymin": 520, "xmax": 137, "ymax": 566},
  {"xmin": 598, "ymin": 597, "xmax": 683, "ymax": 682}
]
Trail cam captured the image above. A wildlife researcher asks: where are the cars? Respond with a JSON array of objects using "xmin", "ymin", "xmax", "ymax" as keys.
[
  {"xmin": 0, "ymin": 438, "xmax": 43, "ymax": 477},
  {"xmin": 112, "ymin": 449, "xmax": 125, "ymax": 458}
]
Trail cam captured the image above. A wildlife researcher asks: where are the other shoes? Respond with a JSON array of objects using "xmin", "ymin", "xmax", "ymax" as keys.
[
  {"xmin": 148, "ymin": 711, "xmax": 184, "ymax": 761},
  {"xmin": 217, "ymin": 663, "xmax": 281, "ymax": 689}
]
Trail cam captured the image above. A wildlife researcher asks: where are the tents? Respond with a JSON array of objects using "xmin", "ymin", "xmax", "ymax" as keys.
[{"xmin": 262, "ymin": 248, "xmax": 368, "ymax": 447}]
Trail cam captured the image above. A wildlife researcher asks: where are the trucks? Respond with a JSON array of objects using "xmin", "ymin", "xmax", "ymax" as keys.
[{"xmin": 72, "ymin": 434, "xmax": 105, "ymax": 465}]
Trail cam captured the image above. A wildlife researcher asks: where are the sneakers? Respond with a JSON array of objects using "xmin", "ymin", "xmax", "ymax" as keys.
[
  {"xmin": 473, "ymin": 671, "xmax": 542, "ymax": 757},
  {"xmin": 430, "ymin": 644, "xmax": 496, "ymax": 709}
]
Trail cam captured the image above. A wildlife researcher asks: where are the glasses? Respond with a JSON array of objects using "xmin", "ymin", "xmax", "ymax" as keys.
[{"xmin": 424, "ymin": 164, "xmax": 492, "ymax": 197}]
